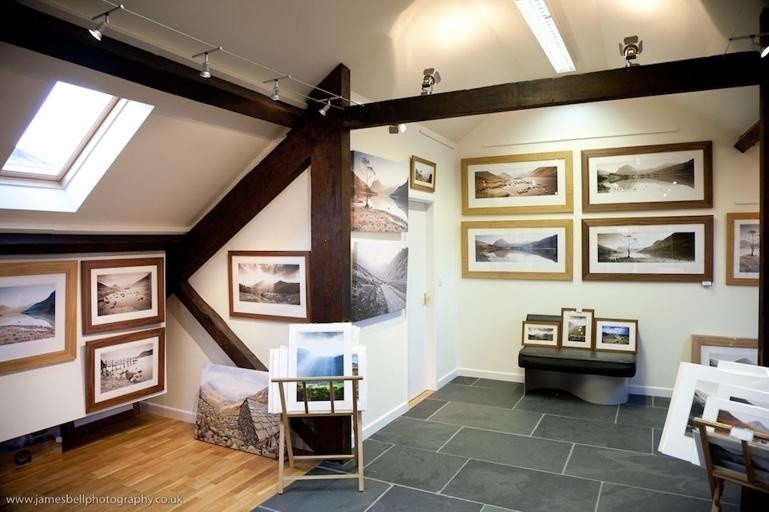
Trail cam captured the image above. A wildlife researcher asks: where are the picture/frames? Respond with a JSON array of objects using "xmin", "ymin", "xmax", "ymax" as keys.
[
  {"xmin": 657, "ymin": 332, "xmax": 768, "ymax": 494},
  {"xmin": 460, "ymin": 150, "xmax": 574, "ymax": 214},
  {"xmin": 81, "ymin": 256, "xmax": 166, "ymax": 335},
  {"xmin": 519, "ymin": 301, "xmax": 641, "ymax": 354},
  {"xmin": 581, "ymin": 214, "xmax": 714, "ymax": 283},
  {"xmin": 460, "ymin": 219, "xmax": 574, "ymax": 281},
  {"xmin": 581, "ymin": 139, "xmax": 713, "ymax": 212},
  {"xmin": 84, "ymin": 325, "xmax": 166, "ymax": 414},
  {"xmin": 724, "ymin": 211, "xmax": 760, "ymax": 286},
  {"xmin": 410, "ymin": 155, "xmax": 437, "ymax": 192},
  {"xmin": 226, "ymin": 250, "xmax": 312, "ymax": 322},
  {"xmin": 0, "ymin": 260, "xmax": 79, "ymax": 375},
  {"xmin": 267, "ymin": 321, "xmax": 368, "ymax": 415}
]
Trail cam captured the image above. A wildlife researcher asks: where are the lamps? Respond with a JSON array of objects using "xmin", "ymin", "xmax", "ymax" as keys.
[
  {"xmin": 618, "ymin": 34, "xmax": 645, "ymax": 70},
  {"xmin": 318, "ymin": 99, "xmax": 333, "ymax": 117},
  {"xmin": 198, "ymin": 53, "xmax": 213, "ymax": 79},
  {"xmin": 422, "ymin": 67, "xmax": 443, "ymax": 98},
  {"xmin": 269, "ymin": 77, "xmax": 281, "ymax": 101},
  {"xmin": 87, "ymin": 12, "xmax": 111, "ymax": 42}
]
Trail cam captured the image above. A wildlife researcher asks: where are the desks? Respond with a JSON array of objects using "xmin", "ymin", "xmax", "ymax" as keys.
[{"xmin": 516, "ymin": 345, "xmax": 637, "ymax": 406}]
